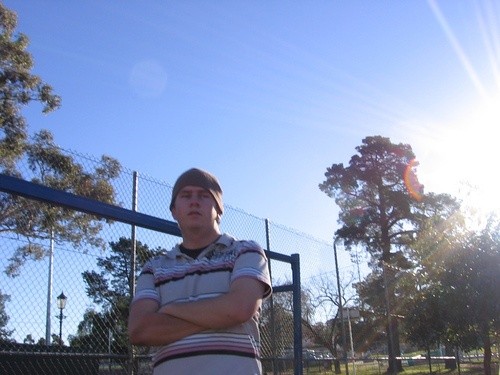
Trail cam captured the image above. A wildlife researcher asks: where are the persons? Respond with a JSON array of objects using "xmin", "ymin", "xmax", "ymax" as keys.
[{"xmin": 128, "ymin": 168, "xmax": 272, "ymax": 375}]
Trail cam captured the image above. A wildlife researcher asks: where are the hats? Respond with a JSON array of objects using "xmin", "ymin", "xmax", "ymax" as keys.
[{"xmin": 169, "ymin": 168, "xmax": 223, "ymax": 215}]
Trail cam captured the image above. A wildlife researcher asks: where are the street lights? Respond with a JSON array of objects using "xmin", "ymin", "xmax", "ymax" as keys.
[{"xmin": 56, "ymin": 290, "xmax": 66, "ymax": 353}]
